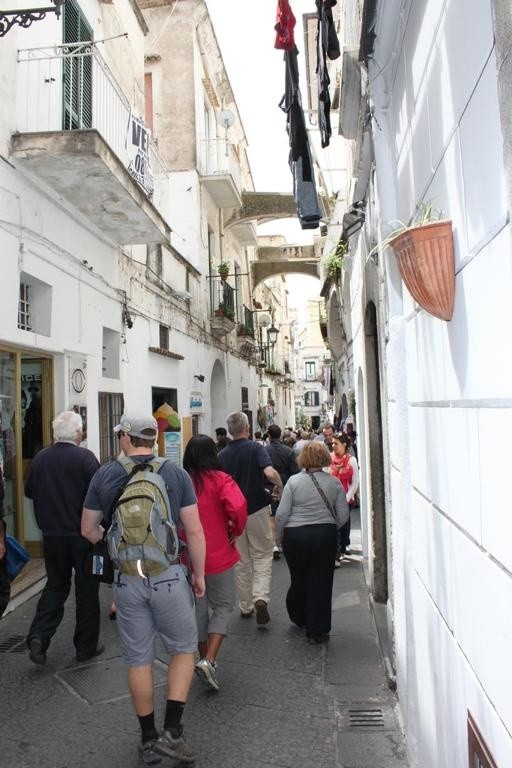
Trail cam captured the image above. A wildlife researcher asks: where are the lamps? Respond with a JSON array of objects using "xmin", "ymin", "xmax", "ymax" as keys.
[{"xmin": 248, "ymin": 322, "xmax": 281, "ymax": 356}]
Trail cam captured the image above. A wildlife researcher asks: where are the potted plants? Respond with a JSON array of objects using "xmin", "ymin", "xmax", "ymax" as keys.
[
  {"xmin": 237, "ymin": 322, "xmax": 254, "ymax": 340},
  {"xmin": 361, "ymin": 193, "xmax": 455, "ymax": 322},
  {"xmin": 324, "ymin": 236, "xmax": 348, "ymax": 285},
  {"xmin": 216, "ymin": 259, "xmax": 231, "ymax": 280},
  {"xmin": 214, "ymin": 301, "xmax": 235, "ymax": 324}
]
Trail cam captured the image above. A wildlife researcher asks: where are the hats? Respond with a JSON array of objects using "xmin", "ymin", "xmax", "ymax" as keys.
[{"xmin": 115, "ymin": 412, "xmax": 160, "ymax": 439}]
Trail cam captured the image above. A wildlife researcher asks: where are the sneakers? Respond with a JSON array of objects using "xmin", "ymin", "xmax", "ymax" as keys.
[
  {"xmin": 29, "ymin": 639, "xmax": 46, "ymax": 666},
  {"xmin": 79, "ymin": 644, "xmax": 104, "ymax": 662},
  {"xmin": 255, "ymin": 599, "xmax": 271, "ymax": 624},
  {"xmin": 153, "ymin": 722, "xmax": 197, "ymax": 763},
  {"xmin": 139, "ymin": 735, "xmax": 162, "ymax": 762},
  {"xmin": 196, "ymin": 659, "xmax": 220, "ymax": 692}
]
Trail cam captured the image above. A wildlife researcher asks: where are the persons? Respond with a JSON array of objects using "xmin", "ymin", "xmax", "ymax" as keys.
[
  {"xmin": 1, "ymin": 468, "xmax": 9, "ymax": 617},
  {"xmin": 81, "ymin": 410, "xmax": 208, "ymax": 767},
  {"xmin": 23, "ymin": 413, "xmax": 104, "ymax": 665}
]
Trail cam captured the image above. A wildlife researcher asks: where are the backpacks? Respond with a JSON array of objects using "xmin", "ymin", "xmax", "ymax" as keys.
[{"xmin": 105, "ymin": 456, "xmax": 179, "ymax": 578}]
